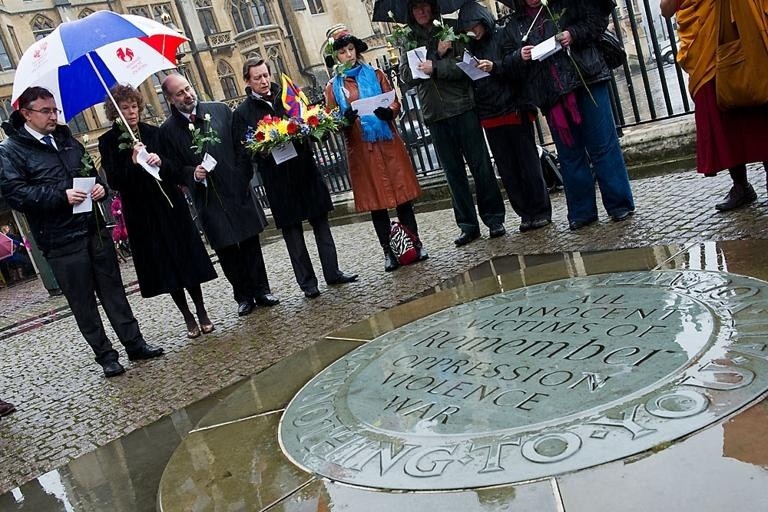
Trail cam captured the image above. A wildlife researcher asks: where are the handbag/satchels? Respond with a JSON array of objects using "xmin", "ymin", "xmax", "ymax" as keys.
[
  {"xmin": 596, "ymin": 29, "xmax": 627, "ymax": 70},
  {"xmin": 389, "ymin": 221, "xmax": 420, "ymax": 267}
]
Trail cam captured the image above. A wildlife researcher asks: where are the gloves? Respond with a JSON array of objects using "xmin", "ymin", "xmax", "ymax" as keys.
[
  {"xmin": 344, "ymin": 106, "xmax": 360, "ymax": 124},
  {"xmin": 373, "ymin": 107, "xmax": 393, "ymax": 121}
]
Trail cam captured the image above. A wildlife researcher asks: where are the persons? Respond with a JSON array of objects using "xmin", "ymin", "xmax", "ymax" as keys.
[
  {"xmin": 159, "ymin": 72, "xmax": 281, "ymax": 317},
  {"xmin": 397, "ymin": 1, "xmax": 506, "ymax": 246},
  {"xmin": 110, "ymin": 190, "xmax": 128, "ymax": 243},
  {"xmin": 456, "ymin": 1, "xmax": 553, "ymax": 230},
  {"xmin": 96, "ymin": 83, "xmax": 219, "ymax": 340},
  {"xmin": 323, "ymin": 24, "xmax": 429, "ymax": 272},
  {"xmin": 659, "ymin": 2, "xmax": 767, "ymax": 211},
  {"xmin": 501, "ymin": 1, "xmax": 636, "ymax": 230},
  {"xmin": 229, "ymin": 56, "xmax": 360, "ymax": 300},
  {"xmin": 1, "ymin": 224, "xmax": 24, "ymax": 282},
  {"xmin": 0, "ymin": 86, "xmax": 163, "ymax": 378}
]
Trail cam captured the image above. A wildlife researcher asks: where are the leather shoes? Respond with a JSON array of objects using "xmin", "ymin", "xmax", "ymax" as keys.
[
  {"xmin": 127, "ymin": 341, "xmax": 163, "ymax": 360},
  {"xmin": 454, "ymin": 228, "xmax": 480, "ymax": 245},
  {"xmin": 238, "ymin": 273, "xmax": 359, "ymax": 315},
  {"xmin": 385, "ymin": 251, "xmax": 398, "ymax": 271},
  {"xmin": 0, "ymin": 398, "xmax": 14, "ymax": 416},
  {"xmin": 715, "ymin": 182, "xmax": 757, "ymax": 211},
  {"xmin": 519, "ymin": 209, "xmax": 630, "ymax": 232},
  {"xmin": 490, "ymin": 223, "xmax": 505, "ymax": 238},
  {"xmin": 103, "ymin": 359, "xmax": 124, "ymax": 376},
  {"xmin": 416, "ymin": 247, "xmax": 428, "ymax": 260}
]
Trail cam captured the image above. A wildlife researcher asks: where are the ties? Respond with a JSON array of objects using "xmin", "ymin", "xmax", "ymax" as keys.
[
  {"xmin": 42, "ymin": 136, "xmax": 55, "ymax": 149},
  {"xmin": 190, "ymin": 114, "xmax": 195, "ymax": 122}
]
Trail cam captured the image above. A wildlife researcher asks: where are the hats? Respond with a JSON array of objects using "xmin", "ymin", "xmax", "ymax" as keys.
[{"xmin": 322, "ymin": 23, "xmax": 368, "ymax": 68}]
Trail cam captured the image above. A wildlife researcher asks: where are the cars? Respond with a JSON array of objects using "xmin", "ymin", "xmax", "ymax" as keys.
[
  {"xmin": 312, "ymin": 146, "xmax": 344, "ymax": 178},
  {"xmin": 651, "ymin": 32, "xmax": 683, "ymax": 65},
  {"xmin": 395, "ymin": 108, "xmax": 433, "ymax": 149}
]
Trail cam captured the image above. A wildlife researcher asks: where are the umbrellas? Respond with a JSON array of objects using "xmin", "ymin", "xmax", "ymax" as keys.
[
  {"xmin": 10, "ymin": 9, "xmax": 193, "ymax": 210},
  {"xmin": 0, "ymin": 232, "xmax": 14, "ymax": 261}
]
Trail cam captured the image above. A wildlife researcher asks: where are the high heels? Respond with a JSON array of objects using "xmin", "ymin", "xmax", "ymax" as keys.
[
  {"xmin": 186, "ymin": 325, "xmax": 201, "ymax": 338},
  {"xmin": 200, "ymin": 321, "xmax": 214, "ymax": 334}
]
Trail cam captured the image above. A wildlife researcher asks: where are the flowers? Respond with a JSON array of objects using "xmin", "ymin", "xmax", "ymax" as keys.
[
  {"xmin": 76, "ymin": 134, "xmax": 106, "ymax": 248},
  {"xmin": 539, "ymin": 0, "xmax": 599, "ymax": 109},
  {"xmin": 240, "ymin": 102, "xmax": 348, "ymax": 157},
  {"xmin": 387, "ymin": 10, "xmax": 443, "ymax": 103},
  {"xmin": 115, "ymin": 117, "xmax": 174, "ymax": 209},
  {"xmin": 188, "ymin": 113, "xmax": 227, "ymax": 213},
  {"xmin": 432, "ymin": 19, "xmax": 480, "ymax": 66},
  {"xmin": 324, "ymin": 36, "xmax": 352, "ymax": 77}
]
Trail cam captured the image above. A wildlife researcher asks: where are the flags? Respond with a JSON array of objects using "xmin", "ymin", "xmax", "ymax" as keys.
[{"xmin": 281, "ymin": 72, "xmax": 309, "ymax": 119}]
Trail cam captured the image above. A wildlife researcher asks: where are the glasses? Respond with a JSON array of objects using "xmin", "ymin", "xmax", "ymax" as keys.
[{"xmin": 25, "ymin": 108, "xmax": 62, "ymax": 116}]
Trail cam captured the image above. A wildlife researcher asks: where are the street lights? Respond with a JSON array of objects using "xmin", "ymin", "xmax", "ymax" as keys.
[{"xmin": 159, "ymin": 5, "xmax": 187, "ymax": 78}]
